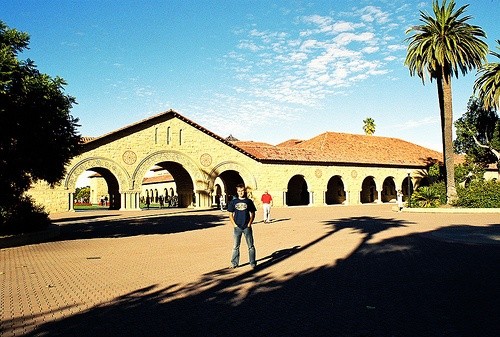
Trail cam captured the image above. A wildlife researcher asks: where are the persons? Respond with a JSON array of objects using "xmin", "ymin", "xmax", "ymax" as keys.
[
  {"xmin": 397, "ymin": 189, "xmax": 404, "ymax": 213},
  {"xmin": 228, "ymin": 182, "xmax": 257, "ymax": 269},
  {"xmin": 159, "ymin": 194, "xmax": 174, "ymax": 207},
  {"xmin": 100, "ymin": 195, "xmax": 109, "ymax": 205},
  {"xmin": 261, "ymin": 190, "xmax": 274, "ymax": 224},
  {"xmin": 146, "ymin": 195, "xmax": 151, "ymax": 210},
  {"xmin": 216, "ymin": 192, "xmax": 221, "ymax": 209}
]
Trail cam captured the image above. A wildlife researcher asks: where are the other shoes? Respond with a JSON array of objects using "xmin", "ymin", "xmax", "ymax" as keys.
[
  {"xmin": 229, "ymin": 265, "xmax": 238, "ymax": 269},
  {"xmin": 252, "ymin": 266, "xmax": 257, "ymax": 269}
]
